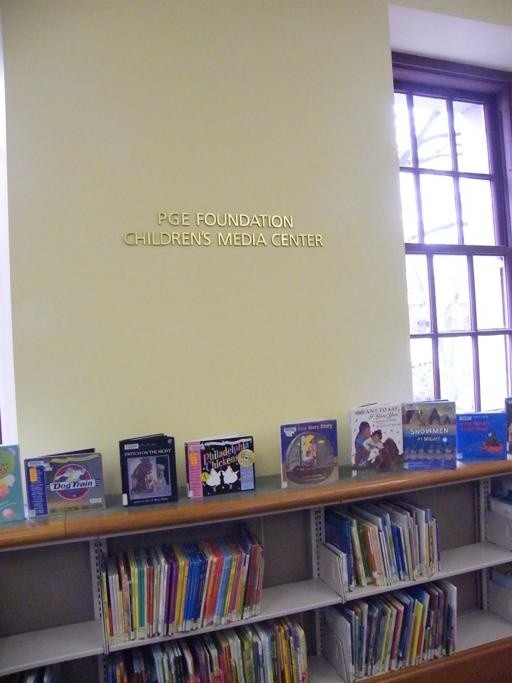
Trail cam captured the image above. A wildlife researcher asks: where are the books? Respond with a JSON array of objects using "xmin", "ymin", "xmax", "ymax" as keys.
[
  {"xmin": 280, "ymin": 417, "xmax": 340, "ymax": 489},
  {"xmin": 0, "ymin": 444, "xmax": 25, "ymax": 524},
  {"xmin": 118, "ymin": 431, "xmax": 178, "ymax": 507},
  {"xmin": 98, "ymin": 530, "xmax": 308, "ymax": 683},
  {"xmin": 24, "ymin": 449, "xmax": 105, "ymax": 518},
  {"xmin": 329, "ymin": 502, "xmax": 458, "ymax": 683},
  {"xmin": 184, "ymin": 433, "xmax": 256, "ymax": 499},
  {"xmin": 350, "ymin": 402, "xmax": 404, "ymax": 477},
  {"xmin": 402, "ymin": 399, "xmax": 457, "ymax": 472},
  {"xmin": 455, "ymin": 409, "xmax": 507, "ymax": 463}
]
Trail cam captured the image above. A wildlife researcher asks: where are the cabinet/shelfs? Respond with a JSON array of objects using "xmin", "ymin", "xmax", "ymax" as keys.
[{"xmin": 1, "ymin": 456, "xmax": 511, "ymax": 683}]
